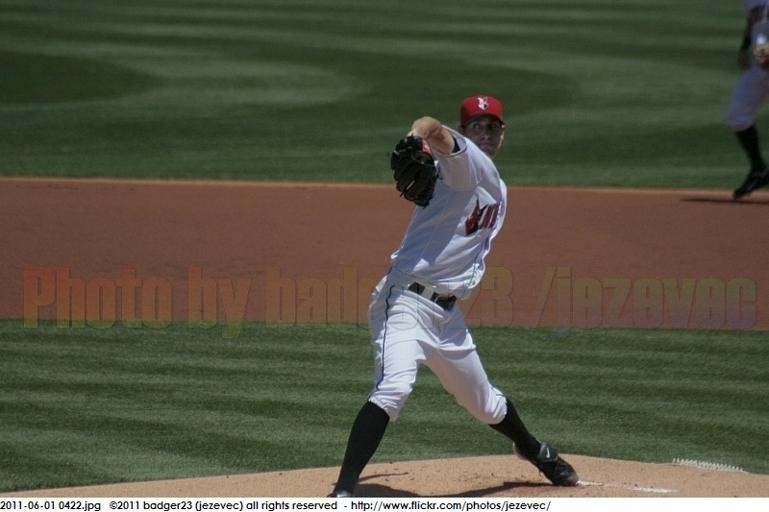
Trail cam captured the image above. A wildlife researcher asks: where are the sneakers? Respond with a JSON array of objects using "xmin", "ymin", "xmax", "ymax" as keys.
[
  {"xmin": 513, "ymin": 439, "xmax": 578, "ymax": 484},
  {"xmin": 734, "ymin": 162, "xmax": 769, "ymax": 199}
]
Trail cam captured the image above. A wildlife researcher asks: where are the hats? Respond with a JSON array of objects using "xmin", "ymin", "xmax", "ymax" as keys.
[{"xmin": 459, "ymin": 95, "xmax": 504, "ymax": 127}]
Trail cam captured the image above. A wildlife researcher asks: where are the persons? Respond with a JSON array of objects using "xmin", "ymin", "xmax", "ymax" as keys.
[
  {"xmin": 325, "ymin": 93, "xmax": 579, "ymax": 499},
  {"xmin": 723, "ymin": 1, "xmax": 769, "ymax": 200}
]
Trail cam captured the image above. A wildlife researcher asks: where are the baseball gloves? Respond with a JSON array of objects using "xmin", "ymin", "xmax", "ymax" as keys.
[{"xmin": 390, "ymin": 134, "xmax": 437, "ymax": 209}]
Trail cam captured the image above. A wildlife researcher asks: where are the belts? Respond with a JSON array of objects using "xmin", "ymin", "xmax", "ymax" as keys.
[{"xmin": 408, "ymin": 282, "xmax": 455, "ymax": 310}]
